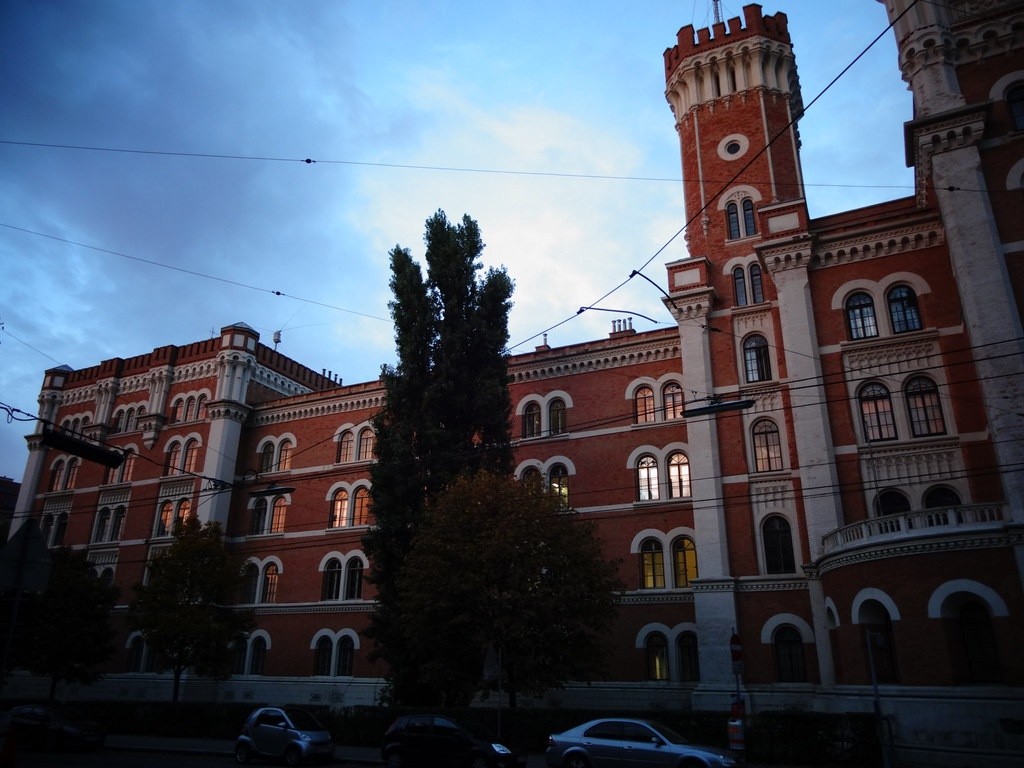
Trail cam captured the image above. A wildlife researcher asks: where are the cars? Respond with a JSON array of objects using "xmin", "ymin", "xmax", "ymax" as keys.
[
  {"xmin": 545, "ymin": 717, "xmax": 744, "ymax": 768},
  {"xmin": 380, "ymin": 713, "xmax": 529, "ymax": 768},
  {"xmin": 234, "ymin": 705, "xmax": 335, "ymax": 762}
]
